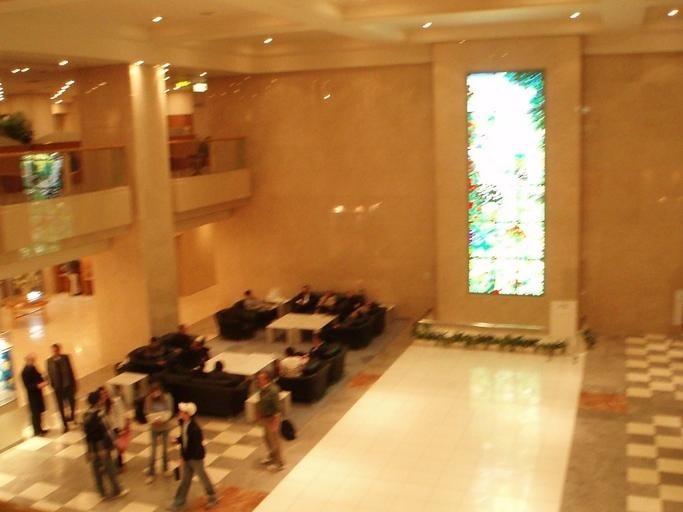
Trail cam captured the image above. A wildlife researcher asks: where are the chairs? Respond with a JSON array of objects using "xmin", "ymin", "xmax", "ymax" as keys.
[
  {"xmin": 128, "ymin": 331, "xmax": 210, "ymax": 371},
  {"xmin": 278, "ymin": 342, "xmax": 348, "ymax": 402},
  {"xmin": 216, "ymin": 299, "xmax": 277, "ymax": 340},
  {"xmin": 324, "ymin": 308, "xmax": 386, "ymax": 349}
]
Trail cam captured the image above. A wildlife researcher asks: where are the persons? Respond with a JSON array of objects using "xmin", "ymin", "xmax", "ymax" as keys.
[
  {"xmin": 278, "ymin": 335, "xmax": 329, "ymax": 377},
  {"xmin": 146, "ymin": 324, "xmax": 231, "ymax": 390},
  {"xmin": 294, "ymin": 285, "xmax": 375, "ymax": 340},
  {"xmin": 189, "ymin": 137, "xmax": 210, "ymax": 174},
  {"xmin": 245, "ymin": 290, "xmax": 265, "ymax": 327},
  {"xmin": 84, "ymin": 386, "xmax": 129, "ymax": 500},
  {"xmin": 257, "ymin": 372, "xmax": 284, "ymax": 471},
  {"xmin": 22, "ymin": 354, "xmax": 49, "ymax": 437},
  {"xmin": 46, "ymin": 343, "xmax": 77, "ymax": 434},
  {"xmin": 170, "ymin": 402, "xmax": 219, "ymax": 512},
  {"xmin": 145, "ymin": 384, "xmax": 173, "ymax": 483}
]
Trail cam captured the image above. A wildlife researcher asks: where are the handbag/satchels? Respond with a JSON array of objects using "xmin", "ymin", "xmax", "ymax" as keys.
[{"xmin": 282, "ymin": 420, "xmax": 295, "ymax": 441}]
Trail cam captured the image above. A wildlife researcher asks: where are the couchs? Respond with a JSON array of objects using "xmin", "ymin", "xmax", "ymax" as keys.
[{"xmin": 156, "ymin": 364, "xmax": 252, "ymax": 416}]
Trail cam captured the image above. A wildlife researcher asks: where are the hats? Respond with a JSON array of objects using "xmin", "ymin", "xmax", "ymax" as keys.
[{"xmin": 178, "ymin": 402, "xmax": 197, "ymax": 417}]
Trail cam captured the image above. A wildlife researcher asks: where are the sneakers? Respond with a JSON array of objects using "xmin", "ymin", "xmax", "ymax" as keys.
[
  {"xmin": 99, "ymin": 488, "xmax": 129, "ymax": 499},
  {"xmin": 35, "ymin": 429, "xmax": 47, "ymax": 436},
  {"xmin": 260, "ymin": 454, "xmax": 284, "ymax": 471}
]
